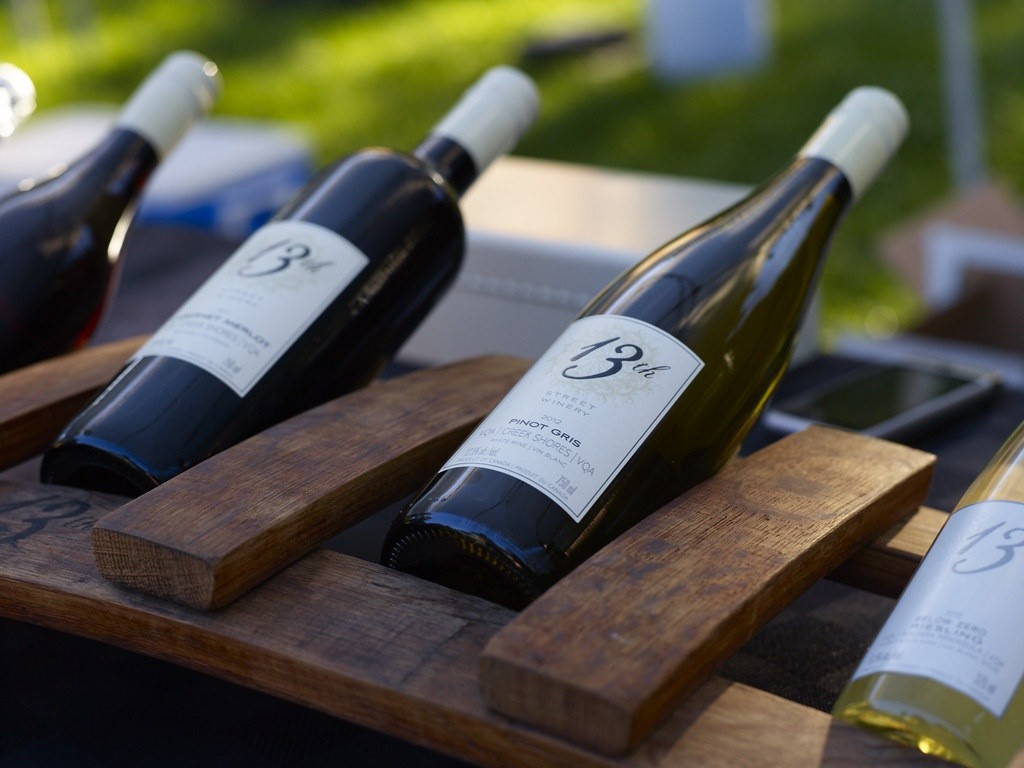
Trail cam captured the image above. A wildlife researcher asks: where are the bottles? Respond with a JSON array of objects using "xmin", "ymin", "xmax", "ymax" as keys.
[
  {"xmin": 39, "ymin": 63, "xmax": 542, "ymax": 496},
  {"xmin": 0, "ymin": 47, "xmax": 223, "ymax": 375},
  {"xmin": 380, "ymin": 85, "xmax": 913, "ymax": 613},
  {"xmin": 830, "ymin": 421, "xmax": 1024, "ymax": 768}
]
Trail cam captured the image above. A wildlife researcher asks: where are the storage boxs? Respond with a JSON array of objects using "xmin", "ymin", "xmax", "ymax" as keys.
[{"xmin": 402, "ymin": 157, "xmax": 748, "ymax": 369}]
